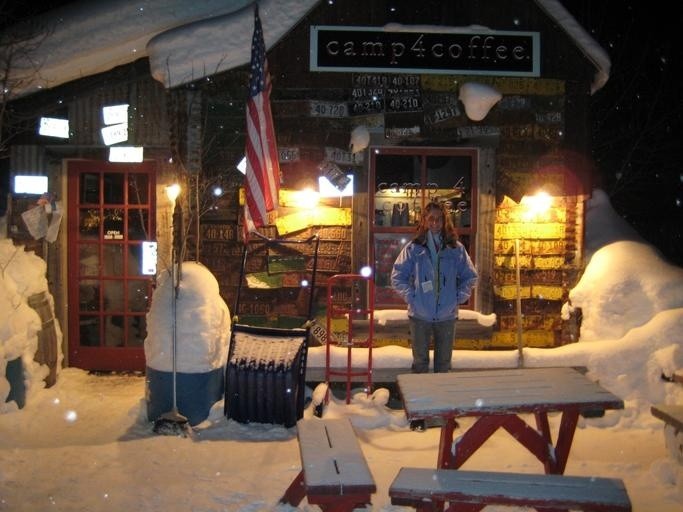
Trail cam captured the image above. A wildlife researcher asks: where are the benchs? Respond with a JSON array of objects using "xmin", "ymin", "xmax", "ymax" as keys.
[
  {"xmin": 651, "ymin": 404, "xmax": 683, "ymax": 463},
  {"xmin": 275, "ymin": 418, "xmax": 377, "ymax": 512},
  {"xmin": 388, "ymin": 468, "xmax": 632, "ymax": 512}
]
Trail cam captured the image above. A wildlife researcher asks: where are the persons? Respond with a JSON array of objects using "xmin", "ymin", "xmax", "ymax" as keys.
[{"xmin": 391, "ymin": 201, "xmax": 478, "ymax": 431}]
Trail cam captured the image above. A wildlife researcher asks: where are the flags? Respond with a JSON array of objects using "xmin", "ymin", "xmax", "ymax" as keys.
[{"xmin": 243, "ymin": 8, "xmax": 281, "ymax": 251}]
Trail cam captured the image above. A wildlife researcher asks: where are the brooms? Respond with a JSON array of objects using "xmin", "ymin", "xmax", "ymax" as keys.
[{"xmin": 152, "ymin": 201, "xmax": 195, "ymax": 439}]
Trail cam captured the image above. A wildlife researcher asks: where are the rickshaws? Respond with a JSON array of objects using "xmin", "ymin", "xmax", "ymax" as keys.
[{"xmin": 222, "ymin": 230, "xmax": 320, "ymax": 428}]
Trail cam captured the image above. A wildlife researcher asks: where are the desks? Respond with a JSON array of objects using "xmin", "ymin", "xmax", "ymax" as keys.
[{"xmin": 396, "ymin": 366, "xmax": 624, "ymax": 475}]
